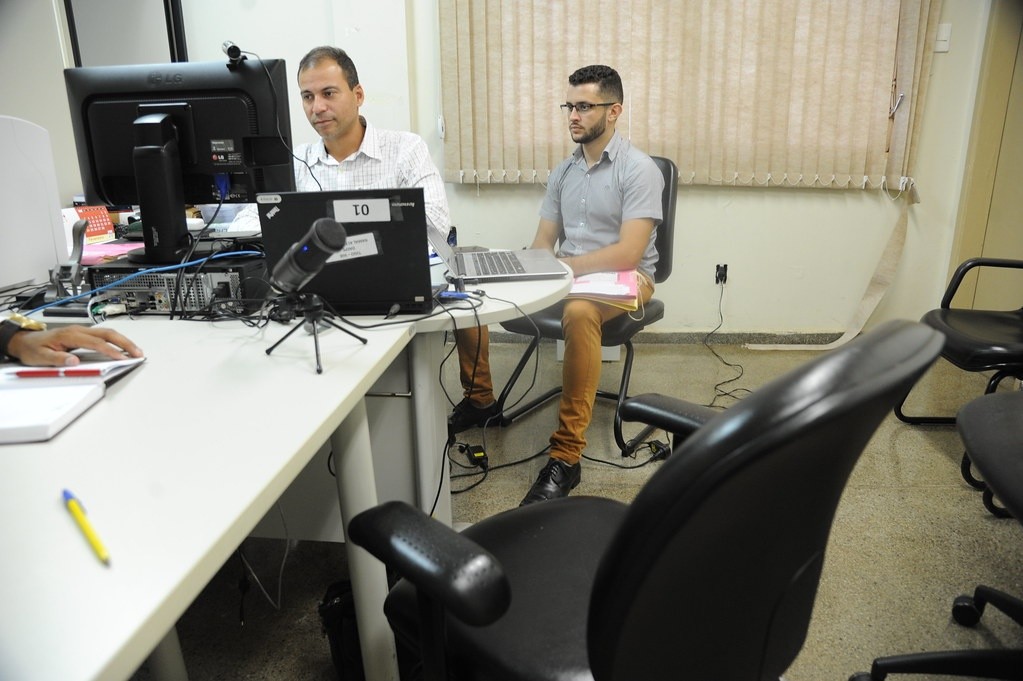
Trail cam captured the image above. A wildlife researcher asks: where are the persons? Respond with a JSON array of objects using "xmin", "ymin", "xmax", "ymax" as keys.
[
  {"xmin": 448, "ymin": 64, "xmax": 665, "ymax": 505},
  {"xmin": 230, "ymin": 47, "xmax": 449, "ymax": 256},
  {"xmin": 0, "ymin": 313, "xmax": 144, "ymax": 366}
]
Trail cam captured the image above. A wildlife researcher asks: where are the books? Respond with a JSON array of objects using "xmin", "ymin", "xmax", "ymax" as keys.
[{"xmin": 0, "ymin": 358, "xmax": 146, "ymax": 445}]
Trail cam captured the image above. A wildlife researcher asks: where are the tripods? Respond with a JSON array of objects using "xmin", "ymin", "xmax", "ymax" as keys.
[{"xmin": 265, "ymin": 295, "xmax": 368, "ymax": 373}]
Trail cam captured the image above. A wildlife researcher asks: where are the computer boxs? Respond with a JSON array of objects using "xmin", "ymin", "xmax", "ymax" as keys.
[{"xmin": 88, "ymin": 246, "xmax": 271, "ymax": 317}]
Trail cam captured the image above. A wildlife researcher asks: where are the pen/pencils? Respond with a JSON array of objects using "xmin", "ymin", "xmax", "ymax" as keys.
[
  {"xmin": 15, "ymin": 370, "xmax": 102, "ymax": 377},
  {"xmin": 63, "ymin": 489, "xmax": 110, "ymax": 568}
]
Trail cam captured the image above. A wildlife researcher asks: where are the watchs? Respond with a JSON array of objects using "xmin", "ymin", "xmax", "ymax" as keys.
[{"xmin": 0, "ymin": 313, "xmax": 47, "ymax": 360}]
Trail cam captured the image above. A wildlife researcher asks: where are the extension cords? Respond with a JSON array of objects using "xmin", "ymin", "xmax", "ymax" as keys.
[{"xmin": 650, "ymin": 439, "xmax": 671, "ymax": 459}]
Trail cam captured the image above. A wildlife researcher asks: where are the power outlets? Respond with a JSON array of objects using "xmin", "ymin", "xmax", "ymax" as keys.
[{"xmin": 715, "ymin": 264, "xmax": 728, "ymax": 286}]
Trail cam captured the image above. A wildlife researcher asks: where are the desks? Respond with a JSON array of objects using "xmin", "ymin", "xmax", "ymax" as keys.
[{"xmin": 1, "ymin": 248, "xmax": 574, "ymax": 681}]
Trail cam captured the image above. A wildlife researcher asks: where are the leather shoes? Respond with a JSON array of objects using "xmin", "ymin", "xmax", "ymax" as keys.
[
  {"xmin": 448, "ymin": 397, "xmax": 500, "ymax": 434},
  {"xmin": 519, "ymin": 458, "xmax": 581, "ymax": 506}
]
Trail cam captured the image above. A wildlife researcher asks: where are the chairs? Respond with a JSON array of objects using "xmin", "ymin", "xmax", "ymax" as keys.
[
  {"xmin": 847, "ymin": 258, "xmax": 1023, "ymax": 681},
  {"xmin": 481, "ymin": 155, "xmax": 678, "ymax": 456},
  {"xmin": 346, "ymin": 319, "xmax": 946, "ymax": 681}
]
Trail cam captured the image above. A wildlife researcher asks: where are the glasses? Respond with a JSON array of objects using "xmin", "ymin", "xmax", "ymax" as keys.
[{"xmin": 560, "ymin": 103, "xmax": 616, "ymax": 115}]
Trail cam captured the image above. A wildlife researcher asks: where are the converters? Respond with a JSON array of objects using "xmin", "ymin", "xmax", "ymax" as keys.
[
  {"xmin": 466, "ymin": 445, "xmax": 488, "ymax": 466},
  {"xmin": 15, "ymin": 288, "xmax": 51, "ymax": 310}
]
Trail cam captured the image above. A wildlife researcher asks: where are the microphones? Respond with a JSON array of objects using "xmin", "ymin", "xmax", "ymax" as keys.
[{"xmin": 262, "ymin": 218, "xmax": 347, "ymax": 308}]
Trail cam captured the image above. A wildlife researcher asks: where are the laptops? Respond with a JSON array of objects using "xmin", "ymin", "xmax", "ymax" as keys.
[
  {"xmin": 425, "ymin": 214, "xmax": 569, "ymax": 284},
  {"xmin": 256, "ymin": 188, "xmax": 447, "ymax": 316}
]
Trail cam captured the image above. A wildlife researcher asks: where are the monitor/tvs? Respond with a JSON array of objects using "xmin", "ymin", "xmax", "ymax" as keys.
[{"xmin": 63, "ymin": 58, "xmax": 296, "ymax": 264}]
